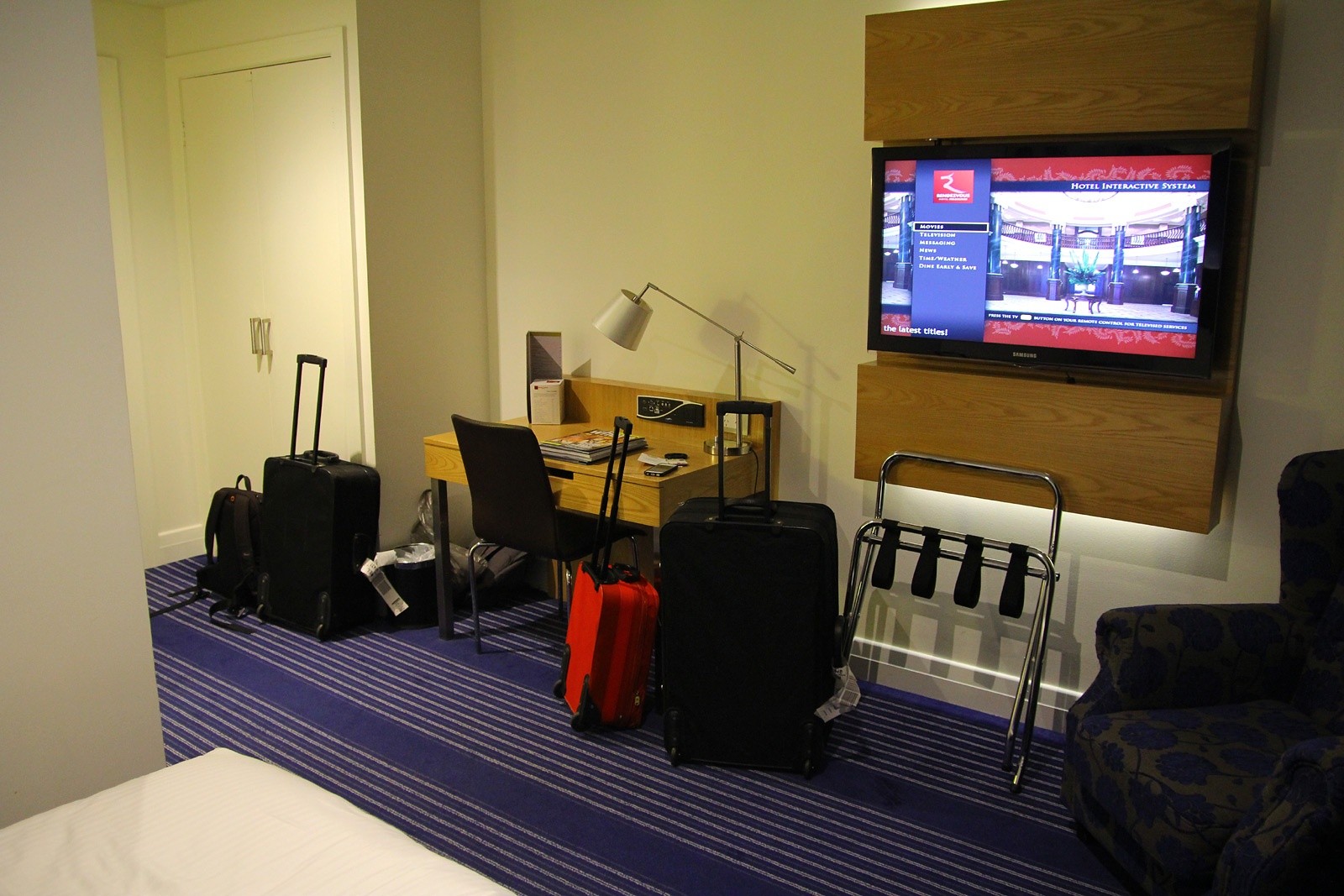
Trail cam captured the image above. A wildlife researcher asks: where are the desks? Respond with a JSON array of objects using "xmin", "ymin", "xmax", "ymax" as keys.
[{"xmin": 424, "ymin": 375, "xmax": 780, "ymax": 641}]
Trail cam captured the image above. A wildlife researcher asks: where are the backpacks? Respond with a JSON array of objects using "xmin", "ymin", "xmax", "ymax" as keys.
[{"xmin": 169, "ymin": 474, "xmax": 263, "ymax": 635}]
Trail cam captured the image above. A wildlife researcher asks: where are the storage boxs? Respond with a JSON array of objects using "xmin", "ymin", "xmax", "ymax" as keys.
[{"xmin": 529, "ymin": 378, "xmax": 565, "ymax": 425}]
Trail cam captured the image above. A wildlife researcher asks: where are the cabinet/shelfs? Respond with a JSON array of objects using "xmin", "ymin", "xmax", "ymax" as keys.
[{"xmin": 90, "ymin": 5, "xmax": 505, "ymax": 557}]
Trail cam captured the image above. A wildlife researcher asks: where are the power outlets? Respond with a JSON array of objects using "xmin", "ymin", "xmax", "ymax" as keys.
[{"xmin": 715, "ymin": 413, "xmax": 749, "ymax": 436}]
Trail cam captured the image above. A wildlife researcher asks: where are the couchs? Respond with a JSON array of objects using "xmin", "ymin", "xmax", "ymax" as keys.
[{"xmin": 1066, "ymin": 450, "xmax": 1343, "ymax": 896}]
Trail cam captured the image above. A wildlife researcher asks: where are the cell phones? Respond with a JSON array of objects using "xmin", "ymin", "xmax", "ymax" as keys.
[{"xmin": 645, "ymin": 463, "xmax": 677, "ymax": 477}]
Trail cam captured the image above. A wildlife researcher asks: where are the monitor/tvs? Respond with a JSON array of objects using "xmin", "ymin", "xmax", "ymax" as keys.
[{"xmin": 867, "ymin": 137, "xmax": 1233, "ymax": 378}]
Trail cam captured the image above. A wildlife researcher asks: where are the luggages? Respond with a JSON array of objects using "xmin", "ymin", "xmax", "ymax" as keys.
[
  {"xmin": 659, "ymin": 401, "xmax": 838, "ymax": 781},
  {"xmin": 257, "ymin": 354, "xmax": 380, "ymax": 640},
  {"xmin": 554, "ymin": 416, "xmax": 657, "ymax": 731}
]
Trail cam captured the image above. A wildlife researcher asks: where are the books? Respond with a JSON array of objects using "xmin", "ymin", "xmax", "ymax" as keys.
[{"xmin": 539, "ymin": 429, "xmax": 648, "ymax": 466}]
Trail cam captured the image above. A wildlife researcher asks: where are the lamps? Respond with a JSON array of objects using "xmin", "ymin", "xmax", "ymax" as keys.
[{"xmin": 593, "ymin": 282, "xmax": 795, "ymax": 455}]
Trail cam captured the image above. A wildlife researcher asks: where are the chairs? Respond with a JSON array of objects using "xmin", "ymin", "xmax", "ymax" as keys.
[
  {"xmin": 843, "ymin": 452, "xmax": 1063, "ymax": 793},
  {"xmin": 451, "ymin": 414, "xmax": 648, "ymax": 655}
]
